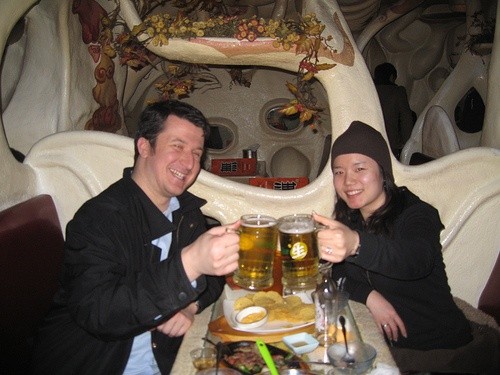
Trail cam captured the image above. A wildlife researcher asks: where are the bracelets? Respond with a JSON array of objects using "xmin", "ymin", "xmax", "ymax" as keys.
[{"xmin": 355, "ymin": 241, "xmax": 361, "ymax": 255}]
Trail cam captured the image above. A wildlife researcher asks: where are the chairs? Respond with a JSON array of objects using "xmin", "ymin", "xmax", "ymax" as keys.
[{"xmin": 0, "ymin": 193, "xmax": 68, "ymax": 330}]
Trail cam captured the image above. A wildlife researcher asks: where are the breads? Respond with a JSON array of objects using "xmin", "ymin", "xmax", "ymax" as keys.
[{"xmin": 267, "ymin": 295, "xmax": 317, "ymax": 323}]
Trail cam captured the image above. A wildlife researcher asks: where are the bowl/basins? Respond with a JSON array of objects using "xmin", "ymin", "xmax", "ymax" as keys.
[
  {"xmin": 327, "ymin": 342, "xmax": 377, "ymax": 374},
  {"xmin": 234, "ymin": 306, "xmax": 268, "ymax": 329},
  {"xmin": 283, "ymin": 331, "xmax": 319, "ymax": 354}
]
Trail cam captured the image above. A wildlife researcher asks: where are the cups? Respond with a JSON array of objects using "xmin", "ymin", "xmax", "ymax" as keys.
[
  {"xmin": 328, "ymin": 367, "xmax": 358, "ymax": 375},
  {"xmin": 190, "ymin": 348, "xmax": 217, "ymax": 370},
  {"xmin": 278, "ymin": 214, "xmax": 333, "ymax": 291},
  {"xmin": 225, "ymin": 214, "xmax": 279, "ymax": 291}
]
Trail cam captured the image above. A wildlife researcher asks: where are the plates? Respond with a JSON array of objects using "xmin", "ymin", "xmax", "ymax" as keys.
[
  {"xmin": 223, "ymin": 292, "xmax": 316, "ymax": 334},
  {"xmin": 218, "ymin": 340, "xmax": 301, "ymax": 375}
]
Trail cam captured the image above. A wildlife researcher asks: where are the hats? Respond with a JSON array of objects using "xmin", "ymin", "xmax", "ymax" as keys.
[{"xmin": 331, "ymin": 121, "xmax": 394, "ymax": 181}]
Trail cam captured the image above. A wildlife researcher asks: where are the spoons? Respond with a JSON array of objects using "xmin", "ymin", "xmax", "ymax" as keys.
[{"xmin": 339, "ymin": 316, "xmax": 355, "ymax": 368}]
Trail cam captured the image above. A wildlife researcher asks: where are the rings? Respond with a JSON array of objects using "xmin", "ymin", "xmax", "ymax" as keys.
[
  {"xmin": 326, "ymin": 247, "xmax": 333, "ymax": 255},
  {"xmin": 383, "ymin": 323, "xmax": 388, "ymax": 328}
]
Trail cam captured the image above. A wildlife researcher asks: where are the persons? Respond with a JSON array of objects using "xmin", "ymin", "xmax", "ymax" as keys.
[
  {"xmin": 311, "ymin": 120, "xmax": 472, "ymax": 375},
  {"xmin": 374, "ymin": 63, "xmax": 412, "ymax": 161},
  {"xmin": 35, "ymin": 101, "xmax": 244, "ymax": 375}
]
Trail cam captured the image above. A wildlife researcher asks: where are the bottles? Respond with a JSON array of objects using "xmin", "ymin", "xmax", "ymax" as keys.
[{"xmin": 311, "ymin": 267, "xmax": 339, "ymax": 347}]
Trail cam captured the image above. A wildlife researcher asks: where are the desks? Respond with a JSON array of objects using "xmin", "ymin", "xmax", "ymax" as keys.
[{"xmin": 169, "ymin": 272, "xmax": 401, "ymax": 375}]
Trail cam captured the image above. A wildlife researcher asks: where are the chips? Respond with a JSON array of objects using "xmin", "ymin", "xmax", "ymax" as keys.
[{"xmin": 234, "ymin": 289, "xmax": 285, "ymax": 310}]
